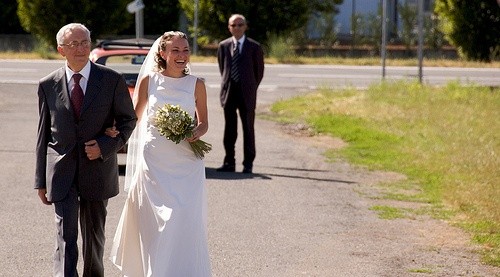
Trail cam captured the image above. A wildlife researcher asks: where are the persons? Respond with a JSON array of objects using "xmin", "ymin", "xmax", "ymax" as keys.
[
  {"xmin": 106, "ymin": 31, "xmax": 211, "ymax": 277},
  {"xmin": 33, "ymin": 22, "xmax": 137, "ymax": 277},
  {"xmin": 216, "ymin": 14, "xmax": 264, "ymax": 174}
]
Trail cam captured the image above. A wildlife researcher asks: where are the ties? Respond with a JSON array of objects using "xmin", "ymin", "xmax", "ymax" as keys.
[
  {"xmin": 231, "ymin": 41, "xmax": 240, "ymax": 82},
  {"xmin": 70, "ymin": 74, "xmax": 85, "ymax": 122}
]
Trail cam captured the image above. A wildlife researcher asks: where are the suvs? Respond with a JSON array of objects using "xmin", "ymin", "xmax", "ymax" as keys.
[{"xmin": 87, "ymin": 39, "xmax": 156, "ymax": 156}]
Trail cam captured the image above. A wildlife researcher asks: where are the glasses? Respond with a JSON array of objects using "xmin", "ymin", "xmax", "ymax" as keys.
[
  {"xmin": 60, "ymin": 40, "xmax": 91, "ymax": 48},
  {"xmin": 230, "ymin": 24, "xmax": 244, "ymax": 28}
]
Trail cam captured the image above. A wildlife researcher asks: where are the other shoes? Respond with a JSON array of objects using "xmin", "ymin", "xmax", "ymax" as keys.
[
  {"xmin": 216, "ymin": 165, "xmax": 235, "ymax": 172},
  {"xmin": 243, "ymin": 167, "xmax": 252, "ymax": 174}
]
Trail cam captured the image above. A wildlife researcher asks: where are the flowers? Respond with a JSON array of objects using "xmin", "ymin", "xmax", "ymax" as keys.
[{"xmin": 152, "ymin": 104, "xmax": 213, "ymax": 159}]
